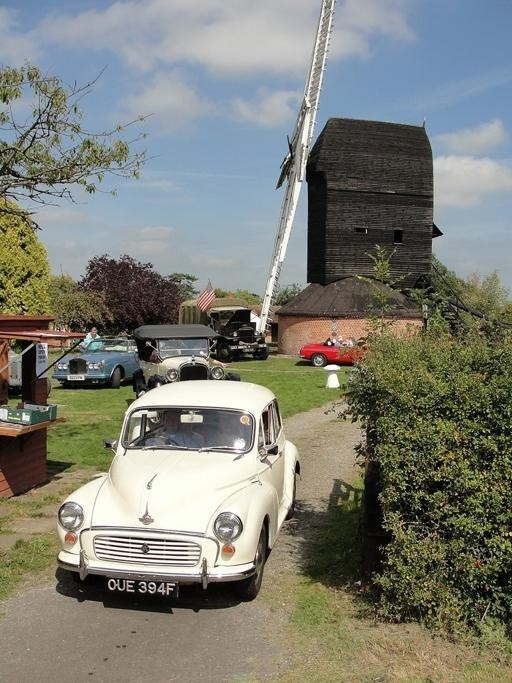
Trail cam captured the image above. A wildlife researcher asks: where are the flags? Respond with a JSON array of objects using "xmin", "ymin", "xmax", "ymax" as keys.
[{"xmin": 197, "ymin": 280, "xmax": 216, "ymax": 313}]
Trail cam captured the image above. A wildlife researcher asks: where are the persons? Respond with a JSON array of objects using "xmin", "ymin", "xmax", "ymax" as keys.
[
  {"xmin": 79, "ymin": 326, "xmax": 103, "ymax": 354},
  {"xmin": 144, "ymin": 409, "xmax": 202, "ymax": 448},
  {"xmin": 334, "ymin": 335, "xmax": 345, "ymax": 346}
]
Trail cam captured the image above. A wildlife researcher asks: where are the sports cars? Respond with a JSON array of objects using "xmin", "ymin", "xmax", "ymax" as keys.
[{"xmin": 299, "ymin": 335, "xmax": 372, "ymax": 368}]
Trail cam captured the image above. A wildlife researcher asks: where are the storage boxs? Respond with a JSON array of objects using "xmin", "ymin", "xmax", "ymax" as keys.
[{"xmin": 0, "ymin": 404, "xmax": 57, "ymax": 426}]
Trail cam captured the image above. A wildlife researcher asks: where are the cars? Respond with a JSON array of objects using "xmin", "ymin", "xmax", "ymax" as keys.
[
  {"xmin": 53, "ymin": 333, "xmax": 140, "ymax": 388},
  {"xmin": 55, "ymin": 376, "xmax": 306, "ymax": 604}
]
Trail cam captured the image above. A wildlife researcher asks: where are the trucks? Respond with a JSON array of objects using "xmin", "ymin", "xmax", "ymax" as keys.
[{"xmin": 178, "ymin": 295, "xmax": 270, "ymax": 361}]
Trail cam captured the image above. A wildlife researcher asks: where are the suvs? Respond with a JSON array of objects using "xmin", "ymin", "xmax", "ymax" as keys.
[
  {"xmin": 1, "ymin": 326, "xmax": 52, "ymax": 401},
  {"xmin": 129, "ymin": 324, "xmax": 241, "ymax": 407}
]
